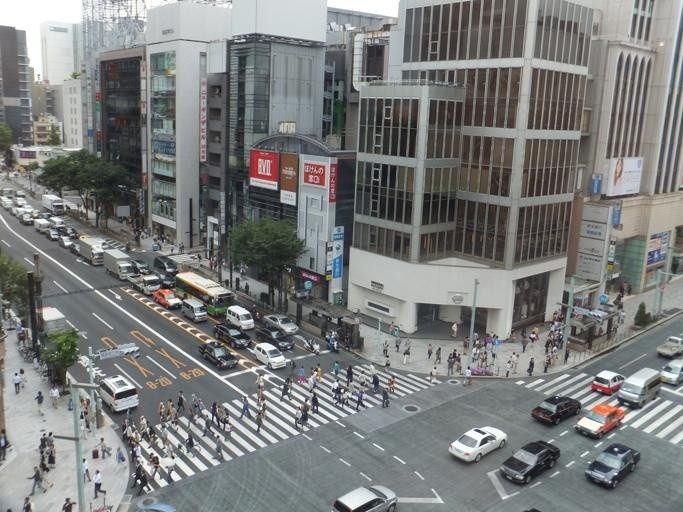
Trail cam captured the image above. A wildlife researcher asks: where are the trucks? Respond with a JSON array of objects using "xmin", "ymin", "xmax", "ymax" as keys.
[{"xmin": 656, "ymin": 336, "xmax": 682, "ymax": 360}]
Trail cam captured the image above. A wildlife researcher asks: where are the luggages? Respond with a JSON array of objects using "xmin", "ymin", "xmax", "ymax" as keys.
[{"xmin": 92, "ymin": 449, "xmax": 98, "ymax": 458}]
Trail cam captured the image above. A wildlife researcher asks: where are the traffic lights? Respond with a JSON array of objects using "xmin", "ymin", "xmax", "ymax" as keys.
[{"xmin": 123, "ymin": 348, "xmax": 140, "ymax": 359}]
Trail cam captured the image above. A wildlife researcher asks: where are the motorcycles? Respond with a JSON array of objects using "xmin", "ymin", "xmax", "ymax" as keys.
[
  {"xmin": 303, "ymin": 343, "xmax": 319, "ymax": 355},
  {"xmin": 325, "ymin": 335, "xmax": 338, "ymax": 353}
]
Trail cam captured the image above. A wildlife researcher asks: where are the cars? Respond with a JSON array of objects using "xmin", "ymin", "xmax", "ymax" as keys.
[
  {"xmin": 659, "ymin": 359, "xmax": 682, "ymax": 386},
  {"xmin": 591, "ymin": 370, "xmax": 624, "ymax": 396},
  {"xmin": 584, "ymin": 443, "xmax": 639, "ymax": 490},
  {"xmin": 574, "ymin": 404, "xmax": 623, "ymax": 440},
  {"xmin": 498, "ymin": 442, "xmax": 559, "ymax": 485},
  {"xmin": 531, "ymin": 396, "xmax": 580, "ymax": 426},
  {"xmin": 447, "ymin": 426, "xmax": 507, "ymax": 463},
  {"xmin": 331, "ymin": 485, "xmax": 397, "ymax": 512}
]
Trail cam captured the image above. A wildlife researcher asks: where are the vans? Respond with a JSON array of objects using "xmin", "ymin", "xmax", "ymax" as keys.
[
  {"xmin": 99, "ymin": 374, "xmax": 139, "ymax": 414},
  {"xmin": 616, "ymin": 368, "xmax": 661, "ymax": 410}
]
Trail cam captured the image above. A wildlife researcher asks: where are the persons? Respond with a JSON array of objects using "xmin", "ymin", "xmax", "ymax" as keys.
[
  {"xmin": 117, "ymin": 354, "xmax": 394, "ymax": 496},
  {"xmin": 1, "ymin": 368, "xmax": 113, "ymax": 511},
  {"xmin": 125, "ymin": 225, "xmax": 219, "ymax": 276},
  {"xmin": 614, "ymin": 281, "xmax": 629, "ymax": 324},
  {"xmin": 326, "ymin": 308, "xmax": 570, "ymax": 386}
]
